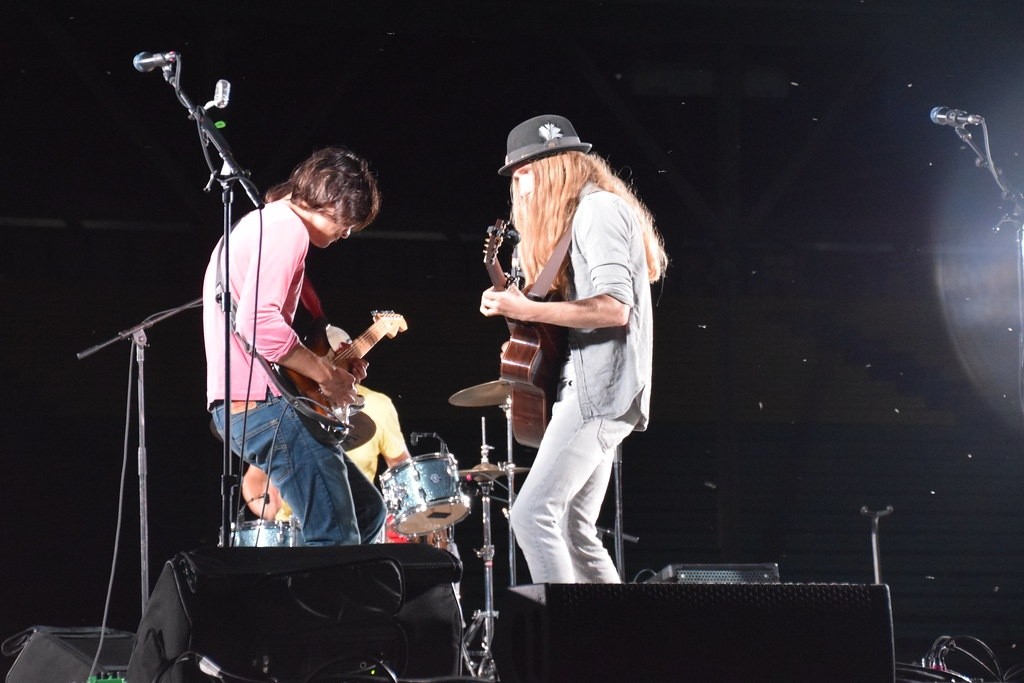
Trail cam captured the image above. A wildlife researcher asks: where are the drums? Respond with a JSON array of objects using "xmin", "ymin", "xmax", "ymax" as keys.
[
  {"xmin": 218, "ymin": 518, "xmax": 296, "ymax": 548},
  {"xmin": 379, "ymin": 452, "xmax": 469, "ymax": 534}
]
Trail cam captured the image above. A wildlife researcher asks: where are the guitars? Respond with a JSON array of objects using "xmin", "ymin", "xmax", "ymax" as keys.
[
  {"xmin": 484, "ymin": 218, "xmax": 572, "ymax": 448},
  {"xmin": 272, "ymin": 310, "xmax": 408, "ymax": 444}
]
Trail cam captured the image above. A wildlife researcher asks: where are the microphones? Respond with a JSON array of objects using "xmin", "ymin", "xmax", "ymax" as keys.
[
  {"xmin": 215, "ymin": 79, "xmax": 230, "ymax": 108},
  {"xmin": 929, "ymin": 106, "xmax": 984, "ymax": 127},
  {"xmin": 132, "ymin": 50, "xmax": 180, "ymax": 72}
]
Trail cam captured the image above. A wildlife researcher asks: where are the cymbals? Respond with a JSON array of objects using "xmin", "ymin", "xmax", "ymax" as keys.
[
  {"xmin": 457, "ymin": 463, "xmax": 529, "ymax": 485},
  {"xmin": 448, "ymin": 380, "xmax": 509, "ymax": 407}
]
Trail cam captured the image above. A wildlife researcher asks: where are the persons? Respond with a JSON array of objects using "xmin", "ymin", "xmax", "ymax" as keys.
[
  {"xmin": 202, "ymin": 145, "xmax": 391, "ymax": 548},
  {"xmin": 480, "ymin": 113, "xmax": 658, "ymax": 582},
  {"xmin": 239, "ymin": 381, "xmax": 422, "ymax": 524}
]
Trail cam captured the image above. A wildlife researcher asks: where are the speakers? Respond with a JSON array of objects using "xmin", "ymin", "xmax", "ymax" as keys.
[
  {"xmin": 498, "ymin": 583, "xmax": 896, "ymax": 683},
  {"xmin": 2, "ymin": 624, "xmax": 137, "ymax": 683},
  {"xmin": 129, "ymin": 543, "xmax": 463, "ymax": 683}
]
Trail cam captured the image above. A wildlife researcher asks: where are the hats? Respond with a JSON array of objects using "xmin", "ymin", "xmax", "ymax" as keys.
[{"xmin": 497, "ymin": 111, "xmax": 592, "ymax": 176}]
